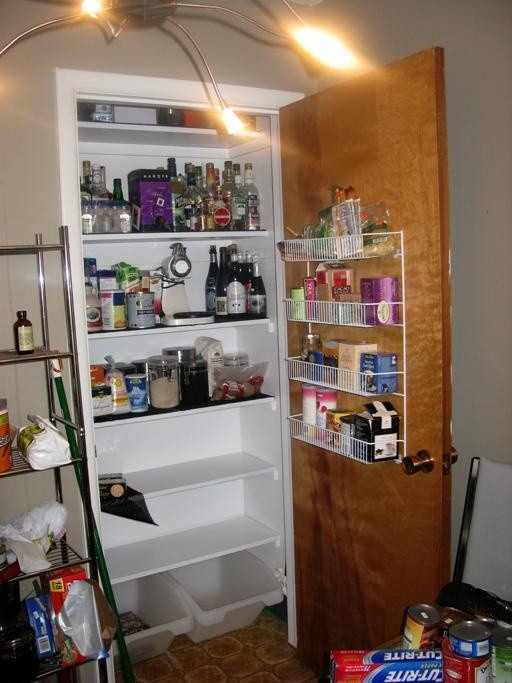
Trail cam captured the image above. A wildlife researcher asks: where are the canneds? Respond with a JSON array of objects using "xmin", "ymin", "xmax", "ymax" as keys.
[
  {"xmin": 126, "ymin": 291, "xmax": 155, "ymax": 329},
  {"xmin": 401, "ymin": 603, "xmax": 512, "ymax": 683}
]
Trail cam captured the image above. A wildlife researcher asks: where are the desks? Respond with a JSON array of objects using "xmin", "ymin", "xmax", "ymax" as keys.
[{"xmin": 319, "ymin": 602, "xmax": 512, "ymax": 683}]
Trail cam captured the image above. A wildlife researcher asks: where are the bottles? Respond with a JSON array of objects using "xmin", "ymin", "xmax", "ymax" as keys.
[
  {"xmin": 80, "ymin": 158, "xmax": 132, "ymax": 234},
  {"xmin": 146, "ymin": 344, "xmax": 248, "ymax": 409},
  {"xmin": 204, "ymin": 244, "xmax": 267, "ymax": 321},
  {"xmin": 12, "ymin": 308, "xmax": 36, "ymax": 353},
  {"xmin": 301, "ymin": 334, "xmax": 337, "ymax": 441},
  {"xmin": 167, "ymin": 155, "xmax": 261, "ymax": 232}
]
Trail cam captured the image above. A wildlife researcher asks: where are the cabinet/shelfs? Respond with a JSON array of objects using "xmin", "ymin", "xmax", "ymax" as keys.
[
  {"xmin": 0, "ymin": 225, "xmax": 108, "ymax": 683},
  {"xmin": 279, "ymin": 227, "xmax": 407, "ymax": 463},
  {"xmin": 39, "ymin": 59, "xmax": 287, "ymax": 673}
]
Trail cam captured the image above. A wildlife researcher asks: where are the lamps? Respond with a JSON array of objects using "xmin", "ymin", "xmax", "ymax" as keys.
[{"xmin": 1, "ymin": 0, "xmax": 364, "ymax": 135}]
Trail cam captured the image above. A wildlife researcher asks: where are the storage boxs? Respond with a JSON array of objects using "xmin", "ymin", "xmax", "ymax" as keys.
[
  {"xmin": 107, "ymin": 575, "xmax": 195, "ymax": 671},
  {"xmin": 166, "ymin": 552, "xmax": 285, "ymax": 643}
]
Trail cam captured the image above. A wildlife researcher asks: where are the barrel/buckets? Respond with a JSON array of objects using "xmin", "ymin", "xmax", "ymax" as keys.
[{"xmin": 435, "ymin": 454, "xmax": 512, "ymax": 623}]
